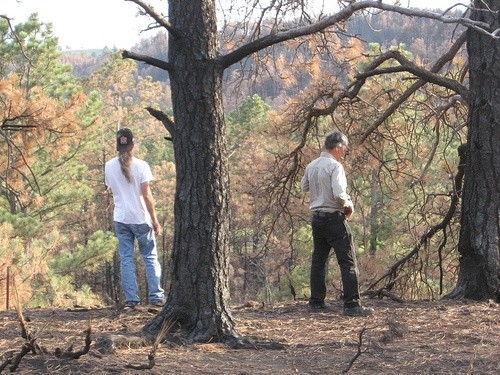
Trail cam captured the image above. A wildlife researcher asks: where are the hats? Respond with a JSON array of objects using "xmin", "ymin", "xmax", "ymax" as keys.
[{"xmin": 116, "ymin": 128, "xmax": 136, "ymax": 152}]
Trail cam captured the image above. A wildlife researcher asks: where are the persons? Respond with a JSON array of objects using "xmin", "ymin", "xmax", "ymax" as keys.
[
  {"xmin": 301, "ymin": 131, "xmax": 374, "ymax": 316},
  {"xmin": 105, "ymin": 128, "xmax": 167, "ymax": 306}
]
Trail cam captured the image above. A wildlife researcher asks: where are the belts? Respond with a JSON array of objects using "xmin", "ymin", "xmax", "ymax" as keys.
[{"xmin": 312, "ymin": 210, "xmax": 346, "ymax": 217}]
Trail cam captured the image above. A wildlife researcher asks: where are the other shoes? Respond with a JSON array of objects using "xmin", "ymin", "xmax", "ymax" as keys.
[
  {"xmin": 125, "ymin": 301, "xmax": 141, "ymax": 305},
  {"xmin": 309, "ymin": 302, "xmax": 336, "ymax": 313},
  {"xmin": 344, "ymin": 303, "xmax": 376, "ymax": 317},
  {"xmin": 148, "ymin": 298, "xmax": 167, "ymax": 306}
]
